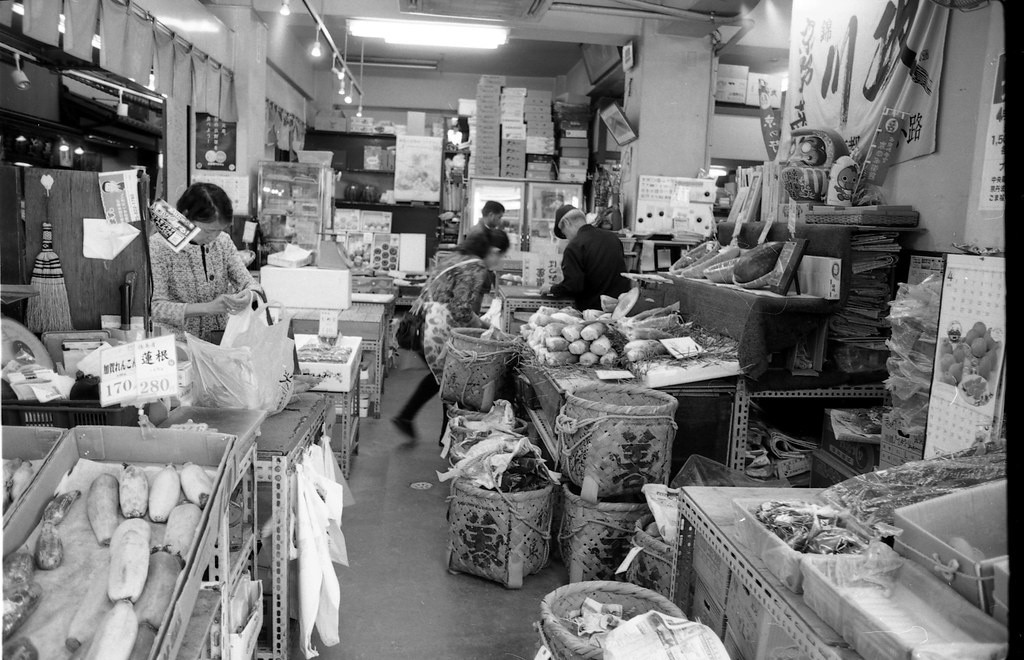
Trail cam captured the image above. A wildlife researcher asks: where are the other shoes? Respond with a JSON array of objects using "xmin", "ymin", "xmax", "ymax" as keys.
[{"xmin": 392, "ymin": 415, "xmax": 415, "ymax": 439}]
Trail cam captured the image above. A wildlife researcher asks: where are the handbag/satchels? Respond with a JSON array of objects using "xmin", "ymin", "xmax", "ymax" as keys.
[
  {"xmin": 396, "ymin": 311, "xmax": 424, "ymax": 352},
  {"xmin": 184, "ymin": 292, "xmax": 303, "ymax": 437}
]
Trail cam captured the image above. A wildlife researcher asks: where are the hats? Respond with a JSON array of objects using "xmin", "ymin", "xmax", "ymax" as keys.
[{"xmin": 554, "ymin": 205, "xmax": 577, "ymax": 239}]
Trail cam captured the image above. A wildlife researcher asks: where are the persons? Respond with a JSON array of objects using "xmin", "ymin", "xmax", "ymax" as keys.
[
  {"xmin": 392, "ymin": 230, "xmax": 509, "ymax": 441},
  {"xmin": 149, "ymin": 183, "xmax": 268, "ymax": 336},
  {"xmin": 467, "ymin": 201, "xmax": 500, "ymax": 242},
  {"xmin": 539, "ymin": 205, "xmax": 630, "ymax": 311}
]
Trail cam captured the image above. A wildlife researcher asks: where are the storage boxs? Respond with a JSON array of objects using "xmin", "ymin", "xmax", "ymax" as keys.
[
  {"xmin": 456, "ymin": 73, "xmax": 589, "ymax": 185},
  {"xmin": 4, "ymin": 425, "xmax": 240, "ymax": 660},
  {"xmin": 731, "ymin": 408, "xmax": 1006, "ymax": 660},
  {"xmin": 293, "ymin": 333, "xmax": 364, "ymax": 391},
  {"xmin": 3, "ymin": 423, "xmax": 70, "ymax": 531},
  {"xmin": 2, "ymin": 397, "xmax": 269, "ymax": 466},
  {"xmin": 715, "ymin": 62, "xmax": 782, "ymax": 110},
  {"xmin": 260, "ymin": 134, "xmax": 443, "ymax": 341}
]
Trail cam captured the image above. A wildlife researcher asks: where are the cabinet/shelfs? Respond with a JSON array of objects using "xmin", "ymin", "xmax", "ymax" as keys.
[
  {"xmin": 303, "ymin": 129, "xmax": 440, "ymax": 270},
  {"xmin": 498, "ymin": 224, "xmax": 928, "ymax": 475},
  {"xmin": 256, "ymin": 162, "xmax": 334, "ymax": 268},
  {"xmin": 672, "ymin": 484, "xmax": 862, "ymax": 659},
  {"xmin": 176, "ymin": 318, "xmax": 391, "ymax": 660}
]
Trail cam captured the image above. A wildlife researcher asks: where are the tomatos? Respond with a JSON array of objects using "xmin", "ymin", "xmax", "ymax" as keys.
[{"xmin": 941, "ymin": 322, "xmax": 997, "ymax": 385}]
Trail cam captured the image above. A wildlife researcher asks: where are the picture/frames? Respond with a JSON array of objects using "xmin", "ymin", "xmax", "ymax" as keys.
[
  {"xmin": 598, "ymin": 101, "xmax": 638, "ymax": 147},
  {"xmin": 579, "ymin": 44, "xmax": 622, "ymax": 85}
]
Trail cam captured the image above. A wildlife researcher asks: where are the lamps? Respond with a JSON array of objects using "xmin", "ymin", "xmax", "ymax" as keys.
[
  {"xmin": 116, "ymin": 91, "xmax": 129, "ymax": 118},
  {"xmin": 281, "ymin": 0, "xmax": 365, "ymax": 120},
  {"xmin": 12, "ymin": 54, "xmax": 30, "ymax": 91}
]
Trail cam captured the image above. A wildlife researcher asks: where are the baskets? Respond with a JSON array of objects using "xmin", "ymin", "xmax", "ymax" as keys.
[
  {"xmin": 670, "ymin": 240, "xmax": 786, "ymax": 288},
  {"xmin": 731, "ymin": 497, "xmax": 879, "ymax": 595},
  {"xmin": 445, "ymin": 384, "xmax": 687, "ymax": 660},
  {"xmin": 439, "ymin": 327, "xmax": 522, "ymax": 412},
  {"xmin": 798, "ymin": 553, "xmax": 1008, "ymax": 660}
]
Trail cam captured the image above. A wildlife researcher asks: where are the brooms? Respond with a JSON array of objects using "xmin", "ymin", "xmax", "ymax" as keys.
[{"xmin": 24, "ymin": 203, "xmax": 75, "ymax": 335}]
